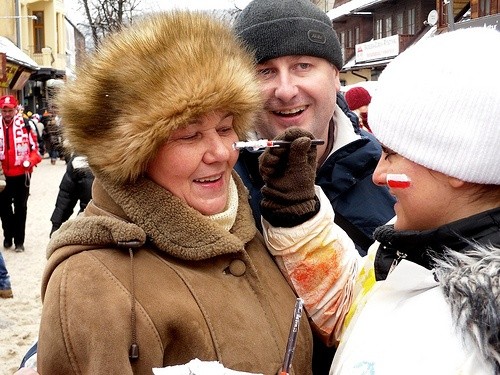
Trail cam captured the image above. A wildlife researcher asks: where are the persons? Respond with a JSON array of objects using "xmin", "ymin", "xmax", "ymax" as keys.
[
  {"xmin": 50, "ymin": 153, "xmax": 94, "ymax": 236},
  {"xmin": 16, "ymin": 104, "xmax": 73, "ymax": 165},
  {"xmin": 0, "ymin": 162, "xmax": 12, "ymax": 298},
  {"xmin": 36, "ymin": 4, "xmax": 313, "ymax": 375},
  {"xmin": 261, "ymin": 27, "xmax": 500, "ymax": 375},
  {"xmin": 341, "ymin": 81, "xmax": 380, "ymax": 132},
  {"xmin": 0, "ymin": 95, "xmax": 43, "ymax": 252},
  {"xmin": 232, "ymin": 1, "xmax": 396, "ymax": 375}
]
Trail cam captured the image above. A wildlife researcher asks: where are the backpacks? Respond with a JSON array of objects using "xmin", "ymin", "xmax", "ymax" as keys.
[{"xmin": 23, "ymin": 118, "xmax": 44, "ymax": 156}]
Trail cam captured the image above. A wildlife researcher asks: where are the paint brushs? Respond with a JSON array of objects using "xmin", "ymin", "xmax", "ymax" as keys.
[
  {"xmin": 281, "ymin": 297, "xmax": 304, "ymax": 372},
  {"xmin": 235, "ymin": 140, "xmax": 325, "ymax": 148}
]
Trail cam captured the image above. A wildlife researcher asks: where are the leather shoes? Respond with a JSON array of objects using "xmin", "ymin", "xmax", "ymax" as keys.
[
  {"xmin": 60, "ymin": 158, "xmax": 65, "ymax": 160},
  {"xmin": 50, "ymin": 159, "xmax": 56, "ymax": 165}
]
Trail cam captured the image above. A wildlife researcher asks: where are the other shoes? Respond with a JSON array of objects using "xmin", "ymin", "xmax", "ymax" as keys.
[
  {"xmin": 0, "ymin": 289, "xmax": 13, "ymax": 299},
  {"xmin": 3, "ymin": 238, "xmax": 25, "ymax": 253}
]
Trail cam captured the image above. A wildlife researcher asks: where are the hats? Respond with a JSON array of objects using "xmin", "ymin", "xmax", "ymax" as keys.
[
  {"xmin": 47, "ymin": 11, "xmax": 268, "ymax": 185},
  {"xmin": 345, "ymin": 87, "xmax": 371, "ymax": 111},
  {"xmin": 0, "ymin": 95, "xmax": 19, "ymax": 109},
  {"xmin": 31, "ymin": 113, "xmax": 40, "ymax": 119},
  {"xmin": 231, "ymin": 0, "xmax": 343, "ymax": 72},
  {"xmin": 367, "ymin": 26, "xmax": 500, "ymax": 184},
  {"xmin": 17, "ymin": 104, "xmax": 24, "ymax": 110}
]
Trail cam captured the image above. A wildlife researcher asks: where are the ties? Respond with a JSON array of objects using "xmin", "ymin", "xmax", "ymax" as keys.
[{"xmin": 3, "ymin": 120, "xmax": 12, "ymax": 148}]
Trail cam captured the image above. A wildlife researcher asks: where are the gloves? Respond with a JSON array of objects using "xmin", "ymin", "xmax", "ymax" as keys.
[{"xmin": 259, "ymin": 126, "xmax": 317, "ymax": 218}]
